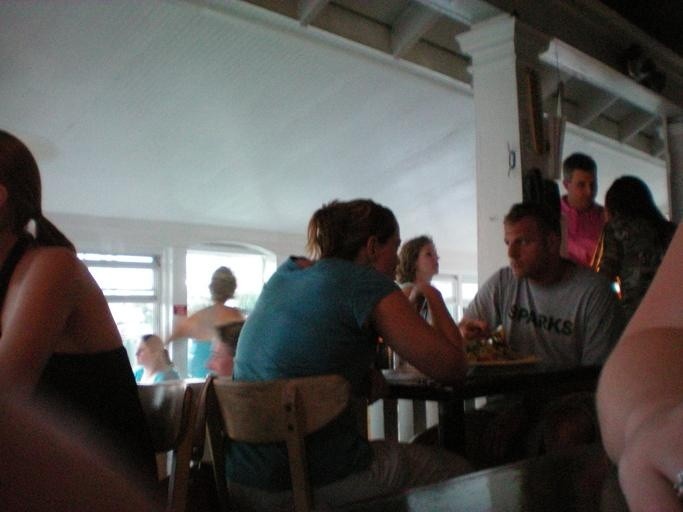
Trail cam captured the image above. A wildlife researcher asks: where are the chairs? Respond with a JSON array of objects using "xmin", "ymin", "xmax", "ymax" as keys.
[
  {"xmin": 130, "ymin": 384, "xmax": 201, "ymax": 511},
  {"xmin": 202, "ymin": 375, "xmax": 351, "ymax": 511}
]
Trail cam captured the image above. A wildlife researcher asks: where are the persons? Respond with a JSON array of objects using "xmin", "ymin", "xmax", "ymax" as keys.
[
  {"xmin": 203, "ymin": 320, "xmax": 243, "ymax": 379},
  {"xmin": 164, "ymin": 267, "xmax": 247, "ymax": 379},
  {"xmin": 410, "ymin": 202, "xmax": 627, "ymax": 511},
  {"xmin": 0, "ymin": 127, "xmax": 162, "ymax": 511},
  {"xmin": 131, "ymin": 335, "xmax": 181, "ymax": 385},
  {"xmin": 225, "ymin": 199, "xmax": 477, "ymax": 512},
  {"xmin": 596, "ymin": 220, "xmax": 682, "ymax": 512},
  {"xmin": 559, "ymin": 153, "xmax": 608, "ymax": 270},
  {"xmin": 590, "ymin": 175, "xmax": 677, "ymax": 319},
  {"xmin": 393, "ymin": 236, "xmax": 439, "ymax": 320}
]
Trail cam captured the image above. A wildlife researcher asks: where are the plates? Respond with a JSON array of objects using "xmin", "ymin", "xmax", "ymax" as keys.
[{"xmin": 469, "ymin": 356, "xmax": 545, "ymax": 371}]
